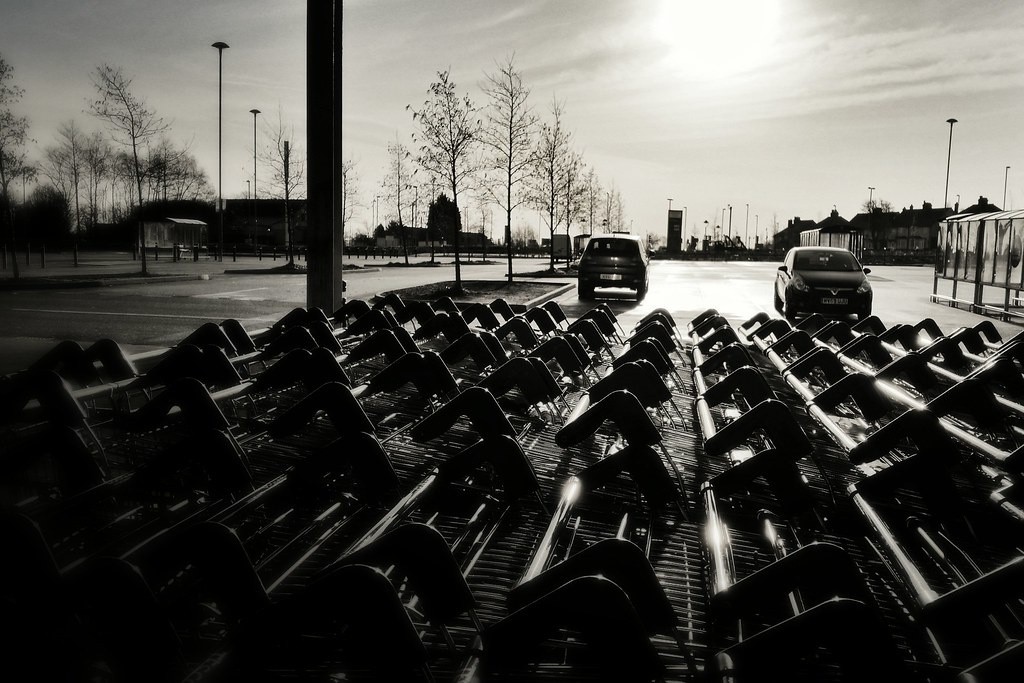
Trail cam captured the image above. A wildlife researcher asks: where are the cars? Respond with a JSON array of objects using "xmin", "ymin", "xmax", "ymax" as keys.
[
  {"xmin": 772, "ymin": 244, "xmax": 874, "ymax": 325},
  {"xmin": 568, "ymin": 232, "xmax": 657, "ymax": 302}
]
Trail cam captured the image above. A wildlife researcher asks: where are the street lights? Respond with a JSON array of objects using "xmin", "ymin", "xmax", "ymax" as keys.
[
  {"xmin": 249, "ymin": 108, "xmax": 261, "ymax": 258},
  {"xmin": 667, "ymin": 196, "xmax": 783, "ymax": 250},
  {"xmin": 212, "ymin": 40, "xmax": 229, "ymax": 263},
  {"xmin": 944, "ymin": 118, "xmax": 958, "ymax": 220},
  {"xmin": 868, "ymin": 186, "xmax": 878, "ymax": 211}
]
